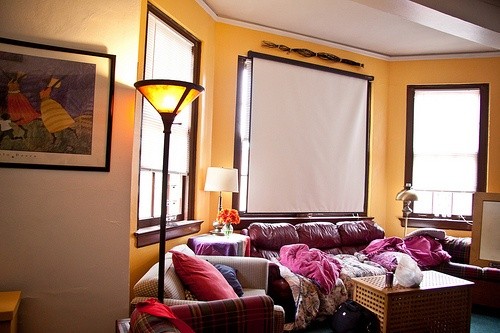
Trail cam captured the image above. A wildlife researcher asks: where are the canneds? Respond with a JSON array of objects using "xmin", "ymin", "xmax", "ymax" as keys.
[{"xmin": 385, "ymin": 272, "xmax": 393, "ymax": 288}]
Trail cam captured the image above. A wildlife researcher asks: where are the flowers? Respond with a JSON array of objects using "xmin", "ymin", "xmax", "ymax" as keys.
[{"xmin": 217, "ymin": 209, "xmax": 240, "ymax": 225}]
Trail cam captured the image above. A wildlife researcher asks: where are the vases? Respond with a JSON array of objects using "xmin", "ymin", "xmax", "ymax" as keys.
[{"xmin": 222, "ymin": 224, "xmax": 233, "ymax": 238}]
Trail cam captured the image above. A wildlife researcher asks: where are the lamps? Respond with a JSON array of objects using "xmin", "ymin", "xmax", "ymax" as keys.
[
  {"xmin": 205, "ymin": 167, "xmax": 238, "ymax": 234},
  {"xmin": 396, "ymin": 184, "xmax": 418, "ymax": 236},
  {"xmin": 134, "ymin": 79, "xmax": 205, "ymax": 303}
]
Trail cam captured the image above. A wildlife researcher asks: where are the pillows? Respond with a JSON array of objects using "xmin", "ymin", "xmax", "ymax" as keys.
[{"xmin": 168, "ymin": 250, "xmax": 244, "ymax": 301}]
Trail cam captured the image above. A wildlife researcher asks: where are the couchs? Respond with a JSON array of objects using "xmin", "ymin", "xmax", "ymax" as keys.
[
  {"xmin": 241, "ymin": 222, "xmax": 386, "ymax": 322},
  {"xmin": 434, "ymin": 236, "xmax": 500, "ymax": 307},
  {"xmin": 130, "ymin": 244, "xmax": 284, "ymax": 333}
]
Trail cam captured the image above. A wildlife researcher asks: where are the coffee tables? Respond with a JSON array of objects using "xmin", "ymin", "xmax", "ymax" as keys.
[{"xmin": 352, "ymin": 270, "xmax": 474, "ymax": 333}]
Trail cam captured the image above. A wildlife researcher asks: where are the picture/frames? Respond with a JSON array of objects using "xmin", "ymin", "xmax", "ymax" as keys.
[{"xmin": 0, "ymin": 37, "xmax": 116, "ymax": 172}]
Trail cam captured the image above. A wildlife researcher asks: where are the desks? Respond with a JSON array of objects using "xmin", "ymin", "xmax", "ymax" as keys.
[
  {"xmin": 0, "ymin": 291, "xmax": 23, "ymax": 333},
  {"xmin": 187, "ymin": 233, "xmax": 250, "ymax": 256}
]
